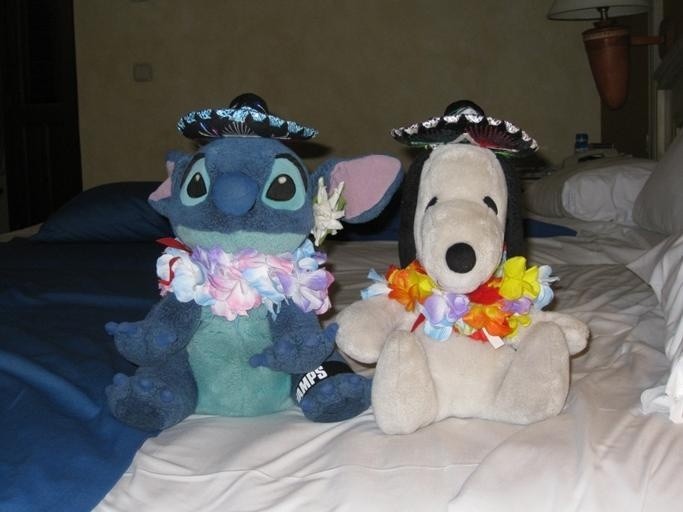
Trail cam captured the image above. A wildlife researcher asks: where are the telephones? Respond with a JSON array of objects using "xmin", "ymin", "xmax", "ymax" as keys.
[{"xmin": 562, "ymin": 147, "xmax": 618, "ymax": 170}]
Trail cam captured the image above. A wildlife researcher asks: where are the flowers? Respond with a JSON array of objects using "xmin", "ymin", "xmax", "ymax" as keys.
[
  {"xmin": 356, "ymin": 251, "xmax": 561, "ymax": 352},
  {"xmin": 152, "ymin": 174, "xmax": 348, "ymax": 324}
]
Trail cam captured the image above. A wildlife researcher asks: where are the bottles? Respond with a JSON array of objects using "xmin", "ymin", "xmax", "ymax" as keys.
[{"xmin": 573, "ymin": 132, "xmax": 589, "ymax": 152}]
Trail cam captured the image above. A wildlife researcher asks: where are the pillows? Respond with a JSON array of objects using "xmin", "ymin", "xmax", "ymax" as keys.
[
  {"xmin": 0, "ymin": 236, "xmax": 167, "ymax": 512},
  {"xmin": 34, "ymin": 178, "xmax": 169, "ymax": 242},
  {"xmin": 628, "ymin": 125, "xmax": 682, "ymax": 236},
  {"xmin": 520, "ymin": 153, "xmax": 658, "ymax": 221}
]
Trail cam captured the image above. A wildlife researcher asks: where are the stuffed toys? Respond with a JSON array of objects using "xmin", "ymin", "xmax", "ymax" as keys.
[
  {"xmin": 102, "ymin": 91, "xmax": 403, "ymax": 432},
  {"xmin": 336, "ymin": 99, "xmax": 592, "ymax": 436}
]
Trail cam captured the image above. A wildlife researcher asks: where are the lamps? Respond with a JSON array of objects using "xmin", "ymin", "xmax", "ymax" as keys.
[{"xmin": 547, "ymin": 0, "xmax": 662, "ymax": 109}]
